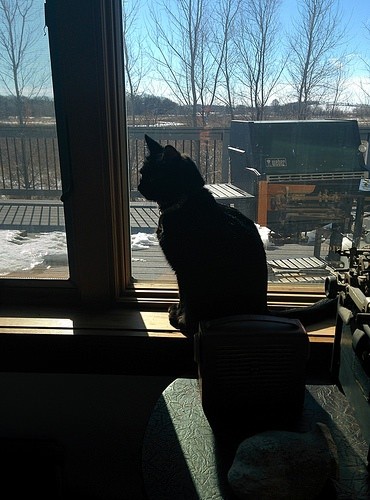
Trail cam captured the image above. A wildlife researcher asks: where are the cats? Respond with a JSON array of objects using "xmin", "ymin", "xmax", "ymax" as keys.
[{"xmin": 130, "ymin": 135, "xmax": 337, "ymax": 337}]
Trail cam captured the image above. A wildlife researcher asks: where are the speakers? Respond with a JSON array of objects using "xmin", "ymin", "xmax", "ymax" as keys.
[{"xmin": 193, "ymin": 313, "xmax": 309, "ymax": 422}]
